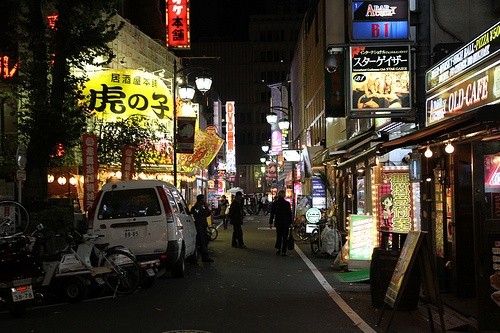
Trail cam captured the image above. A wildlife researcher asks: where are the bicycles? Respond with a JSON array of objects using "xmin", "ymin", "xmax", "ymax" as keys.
[
  {"xmin": 207, "ymin": 225, "xmax": 219, "ymax": 241},
  {"xmin": 56, "ymin": 230, "xmax": 143, "ymax": 298},
  {"xmin": 310, "ymin": 208, "xmax": 332, "ymax": 256},
  {"xmin": 0, "ymin": 200, "xmax": 30, "ymax": 241}
]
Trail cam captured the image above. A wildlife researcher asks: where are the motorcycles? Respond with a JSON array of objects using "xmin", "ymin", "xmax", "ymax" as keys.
[{"xmin": 0, "ymin": 223, "xmax": 49, "ymax": 314}]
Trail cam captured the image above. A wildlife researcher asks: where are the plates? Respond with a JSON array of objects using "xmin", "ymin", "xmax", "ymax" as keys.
[
  {"xmin": 491, "ymin": 291, "xmax": 500, "ymax": 306},
  {"xmin": 490, "ymin": 275, "xmax": 500, "ymax": 290}
]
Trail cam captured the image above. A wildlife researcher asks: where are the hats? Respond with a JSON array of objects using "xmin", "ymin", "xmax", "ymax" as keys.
[{"xmin": 236, "ymin": 191, "xmax": 243, "ymax": 195}]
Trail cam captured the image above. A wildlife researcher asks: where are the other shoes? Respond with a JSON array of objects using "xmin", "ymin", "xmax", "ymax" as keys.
[
  {"xmin": 276, "ymin": 249, "xmax": 280, "ymax": 255},
  {"xmin": 282, "ymin": 252, "xmax": 287, "ymax": 255},
  {"xmin": 202, "ymin": 257, "xmax": 214, "ymax": 262},
  {"xmin": 231, "ymin": 243, "xmax": 246, "ymax": 248}
]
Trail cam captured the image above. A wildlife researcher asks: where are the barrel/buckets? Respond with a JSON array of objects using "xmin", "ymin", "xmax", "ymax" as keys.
[{"xmin": 369, "ymin": 247, "xmax": 421, "ymax": 311}]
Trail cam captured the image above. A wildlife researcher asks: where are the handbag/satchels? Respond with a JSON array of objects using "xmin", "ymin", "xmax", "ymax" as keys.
[{"xmin": 288, "ymin": 228, "xmax": 294, "ymax": 250}]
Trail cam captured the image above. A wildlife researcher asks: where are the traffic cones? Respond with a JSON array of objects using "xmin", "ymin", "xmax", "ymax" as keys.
[{"xmin": 291, "ymin": 214, "xmax": 311, "ymax": 241}]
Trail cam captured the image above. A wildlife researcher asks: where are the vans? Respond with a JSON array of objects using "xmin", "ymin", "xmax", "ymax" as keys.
[{"xmin": 91, "ymin": 178, "xmax": 197, "ymax": 278}]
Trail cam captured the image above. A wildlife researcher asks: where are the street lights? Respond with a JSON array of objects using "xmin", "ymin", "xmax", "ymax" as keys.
[
  {"xmin": 265, "ymin": 105, "xmax": 296, "ymax": 214},
  {"xmin": 171, "ymin": 58, "xmax": 212, "ymax": 188}
]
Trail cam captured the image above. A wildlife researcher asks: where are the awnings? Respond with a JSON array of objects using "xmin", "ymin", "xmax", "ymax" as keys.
[{"xmin": 328, "ymin": 99, "xmax": 500, "ymax": 154}]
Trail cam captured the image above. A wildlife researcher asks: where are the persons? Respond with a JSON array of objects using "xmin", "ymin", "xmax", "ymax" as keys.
[
  {"xmin": 191, "ymin": 195, "xmax": 214, "ymax": 264},
  {"xmin": 357, "ymin": 75, "xmax": 403, "ymax": 108},
  {"xmin": 218, "ymin": 190, "xmax": 293, "ymax": 257}
]
